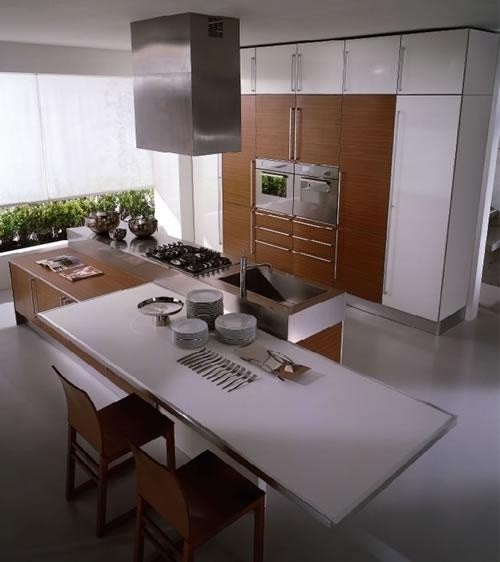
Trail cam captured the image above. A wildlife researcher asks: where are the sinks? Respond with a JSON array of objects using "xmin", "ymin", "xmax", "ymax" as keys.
[{"xmin": 216, "ymin": 265, "xmax": 329, "ymax": 308}]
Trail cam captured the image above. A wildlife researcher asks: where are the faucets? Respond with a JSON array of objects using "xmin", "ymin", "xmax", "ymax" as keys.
[{"xmin": 234, "ymin": 257, "xmax": 276, "ymax": 299}]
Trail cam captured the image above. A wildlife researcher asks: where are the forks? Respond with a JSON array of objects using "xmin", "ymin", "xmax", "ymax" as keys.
[{"xmin": 202, "ymin": 360, "xmax": 257, "ymax": 393}]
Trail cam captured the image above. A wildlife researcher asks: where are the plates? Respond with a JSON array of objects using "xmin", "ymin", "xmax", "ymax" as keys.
[{"xmin": 170, "ymin": 288, "xmax": 257, "ymax": 349}]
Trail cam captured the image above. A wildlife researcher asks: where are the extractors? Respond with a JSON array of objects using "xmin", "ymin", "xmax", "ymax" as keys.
[{"xmin": 130, "ymin": 13, "xmax": 240, "ymax": 156}]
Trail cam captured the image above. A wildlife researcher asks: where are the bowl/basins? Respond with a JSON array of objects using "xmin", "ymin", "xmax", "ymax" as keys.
[{"xmin": 109, "ymin": 228, "xmax": 127, "ymax": 241}]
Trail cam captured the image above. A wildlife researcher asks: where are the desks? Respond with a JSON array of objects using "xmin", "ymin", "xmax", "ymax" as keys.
[{"xmin": 35, "ymin": 282, "xmax": 457, "ymax": 530}]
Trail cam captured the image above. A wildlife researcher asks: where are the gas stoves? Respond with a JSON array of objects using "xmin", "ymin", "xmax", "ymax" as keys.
[{"xmin": 140, "ymin": 240, "xmax": 240, "ymax": 277}]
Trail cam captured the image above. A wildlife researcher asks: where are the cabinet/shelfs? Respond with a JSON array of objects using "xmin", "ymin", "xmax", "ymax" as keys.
[
  {"xmin": 382, "ymin": 95, "xmax": 493, "ymax": 336},
  {"xmin": 343, "ymin": 33, "xmax": 401, "ymax": 98},
  {"xmin": 8, "ymin": 247, "xmax": 164, "ymax": 402},
  {"xmin": 221, "ymin": 96, "xmax": 397, "ymax": 304},
  {"xmin": 240, "ymin": 46, "xmax": 255, "ymax": 96},
  {"xmin": 397, "ymin": 26, "xmax": 500, "ymax": 96},
  {"xmin": 34, "ymin": 277, "xmax": 106, "ymax": 378},
  {"xmin": 255, "ymin": 38, "xmax": 344, "ymax": 95}
]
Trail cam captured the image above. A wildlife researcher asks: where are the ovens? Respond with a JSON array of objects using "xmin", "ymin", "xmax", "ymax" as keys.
[{"xmin": 255, "ymin": 159, "xmax": 339, "ymax": 225}]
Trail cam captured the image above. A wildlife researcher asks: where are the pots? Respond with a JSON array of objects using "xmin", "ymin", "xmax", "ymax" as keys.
[
  {"xmin": 85, "ymin": 210, "xmax": 121, "ymax": 235},
  {"xmin": 127, "ymin": 213, "xmax": 158, "ymax": 237}
]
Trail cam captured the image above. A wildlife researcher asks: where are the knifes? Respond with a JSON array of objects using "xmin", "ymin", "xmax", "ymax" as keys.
[{"xmin": 176, "ymin": 348, "xmax": 226, "ymax": 374}]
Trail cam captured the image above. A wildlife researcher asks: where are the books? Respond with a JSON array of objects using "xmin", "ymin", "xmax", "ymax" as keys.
[
  {"xmin": 35, "ymin": 254, "xmax": 86, "ymax": 272},
  {"xmin": 58, "ymin": 263, "xmax": 104, "ymax": 281}
]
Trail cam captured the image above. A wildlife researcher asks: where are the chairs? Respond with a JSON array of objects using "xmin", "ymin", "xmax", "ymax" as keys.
[
  {"xmin": 129, "ymin": 441, "xmax": 266, "ymax": 562},
  {"xmin": 51, "ymin": 366, "xmax": 176, "ymax": 538}
]
